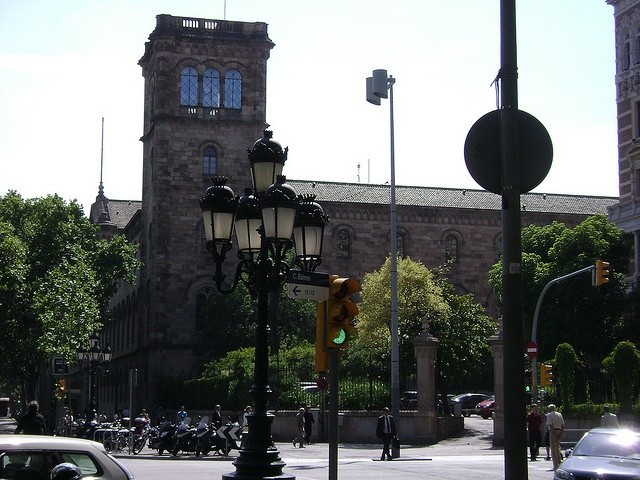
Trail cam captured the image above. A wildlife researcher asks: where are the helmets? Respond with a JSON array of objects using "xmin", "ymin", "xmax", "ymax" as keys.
[{"xmin": 49, "ymin": 463, "xmax": 82, "ymax": 480}]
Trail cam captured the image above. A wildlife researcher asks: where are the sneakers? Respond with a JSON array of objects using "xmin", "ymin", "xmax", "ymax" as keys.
[
  {"xmin": 387, "ymin": 454, "xmax": 392, "ymax": 458},
  {"xmin": 381, "ymin": 454, "xmax": 385, "ymax": 460},
  {"xmin": 292, "ymin": 440, "xmax": 296, "ymax": 447},
  {"xmin": 299, "ymin": 445, "xmax": 306, "ymax": 448}
]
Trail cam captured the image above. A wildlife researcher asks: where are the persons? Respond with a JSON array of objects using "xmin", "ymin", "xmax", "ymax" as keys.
[
  {"xmin": 113, "ymin": 406, "xmax": 129, "ymax": 421},
  {"xmin": 376, "ymin": 407, "xmax": 397, "ymax": 460},
  {"xmin": 98, "ymin": 411, "xmax": 108, "ymax": 422},
  {"xmin": 91, "ymin": 409, "xmax": 99, "ymax": 422},
  {"xmin": 63, "ymin": 410, "xmax": 74, "ymax": 436},
  {"xmin": 155, "ymin": 407, "xmax": 163, "ymax": 424},
  {"xmin": 544, "ymin": 414, "xmax": 563, "ymax": 460},
  {"xmin": 13, "ymin": 401, "xmax": 47, "ymax": 435},
  {"xmin": 228, "ymin": 409, "xmax": 240, "ymax": 423},
  {"xmin": 292, "ymin": 407, "xmax": 306, "ymax": 448},
  {"xmin": 212, "ymin": 405, "xmax": 222, "ymax": 429},
  {"xmin": 239, "ymin": 406, "xmax": 251, "ymax": 449},
  {"xmin": 526, "ymin": 404, "xmax": 542, "ymax": 460},
  {"xmin": 304, "ymin": 404, "xmax": 314, "ymax": 445},
  {"xmin": 138, "ymin": 408, "xmax": 148, "ymax": 419},
  {"xmin": 600, "ymin": 407, "xmax": 620, "ymax": 428},
  {"xmin": 547, "ymin": 404, "xmax": 565, "ymax": 470},
  {"xmin": 177, "ymin": 404, "xmax": 187, "ymax": 425}
]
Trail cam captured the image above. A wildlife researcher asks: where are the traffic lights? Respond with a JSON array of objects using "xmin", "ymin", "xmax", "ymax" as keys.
[
  {"xmin": 58, "ymin": 380, "xmax": 65, "ymax": 396},
  {"xmin": 540, "ymin": 363, "xmax": 553, "ymax": 387},
  {"xmin": 525, "ymin": 371, "xmax": 532, "ymax": 392},
  {"xmin": 596, "ymin": 260, "xmax": 609, "ymax": 286},
  {"xmin": 326, "ymin": 275, "xmax": 360, "ymax": 348}
]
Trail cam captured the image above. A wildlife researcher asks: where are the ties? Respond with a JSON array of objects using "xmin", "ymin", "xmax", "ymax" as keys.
[{"xmin": 386, "ymin": 416, "xmax": 390, "ymax": 433}]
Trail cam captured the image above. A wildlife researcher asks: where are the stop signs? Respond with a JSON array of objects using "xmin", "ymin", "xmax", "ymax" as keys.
[{"xmin": 524, "ymin": 342, "xmax": 538, "ymax": 358}]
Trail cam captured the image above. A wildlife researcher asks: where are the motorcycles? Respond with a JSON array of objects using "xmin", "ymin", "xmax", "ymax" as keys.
[
  {"xmin": 196, "ymin": 416, "xmax": 231, "ymax": 457},
  {"xmin": 159, "ymin": 417, "xmax": 178, "ymax": 455},
  {"xmin": 172, "ymin": 416, "xmax": 198, "ymax": 456},
  {"xmin": 133, "ymin": 418, "xmax": 159, "ymax": 455}
]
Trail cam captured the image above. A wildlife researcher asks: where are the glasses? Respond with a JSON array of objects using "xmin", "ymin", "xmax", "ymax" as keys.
[{"xmin": 387, "ymin": 411, "xmax": 389, "ymax": 412}]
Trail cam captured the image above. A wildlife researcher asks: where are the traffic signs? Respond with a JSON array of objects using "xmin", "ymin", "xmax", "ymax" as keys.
[{"xmin": 287, "ymin": 269, "xmax": 329, "ymax": 300}]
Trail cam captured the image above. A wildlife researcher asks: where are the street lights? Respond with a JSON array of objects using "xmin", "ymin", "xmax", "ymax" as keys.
[
  {"xmin": 366, "ymin": 69, "xmax": 400, "ymax": 457},
  {"xmin": 75, "ymin": 331, "xmax": 112, "ymax": 419},
  {"xmin": 199, "ymin": 130, "xmax": 331, "ymax": 480}
]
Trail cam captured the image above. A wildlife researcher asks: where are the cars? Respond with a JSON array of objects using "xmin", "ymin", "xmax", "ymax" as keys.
[
  {"xmin": 553, "ymin": 427, "xmax": 640, "ymax": 480},
  {"xmin": 401, "ymin": 391, "xmax": 417, "ymax": 409},
  {"xmin": 451, "ymin": 394, "xmax": 490, "ymax": 417},
  {"xmin": 0, "ymin": 434, "xmax": 134, "ymax": 480},
  {"xmin": 475, "ymin": 394, "xmax": 496, "ymax": 419},
  {"xmin": 437, "ymin": 394, "xmax": 455, "ymax": 415}
]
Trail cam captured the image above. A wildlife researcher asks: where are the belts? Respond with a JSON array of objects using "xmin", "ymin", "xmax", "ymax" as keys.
[{"xmin": 549, "ymin": 428, "xmax": 561, "ymax": 432}]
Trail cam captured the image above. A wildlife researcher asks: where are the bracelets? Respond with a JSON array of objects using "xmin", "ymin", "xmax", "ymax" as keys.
[{"xmin": 104, "ymin": 417, "xmax": 105, "ymax": 418}]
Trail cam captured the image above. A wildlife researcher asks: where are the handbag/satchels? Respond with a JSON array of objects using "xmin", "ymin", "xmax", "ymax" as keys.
[{"xmin": 392, "ymin": 435, "xmax": 400, "ymax": 457}]
